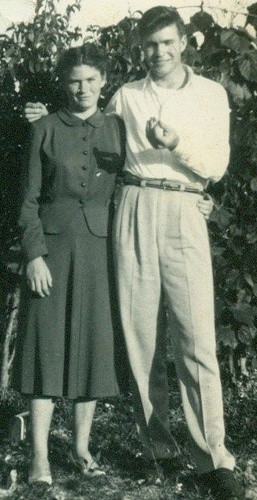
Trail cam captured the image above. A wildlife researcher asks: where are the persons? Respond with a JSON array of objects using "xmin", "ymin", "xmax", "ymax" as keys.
[
  {"xmin": 16, "ymin": 46, "xmax": 214, "ymax": 495},
  {"xmin": 24, "ymin": 7, "xmax": 246, "ymax": 500}
]
